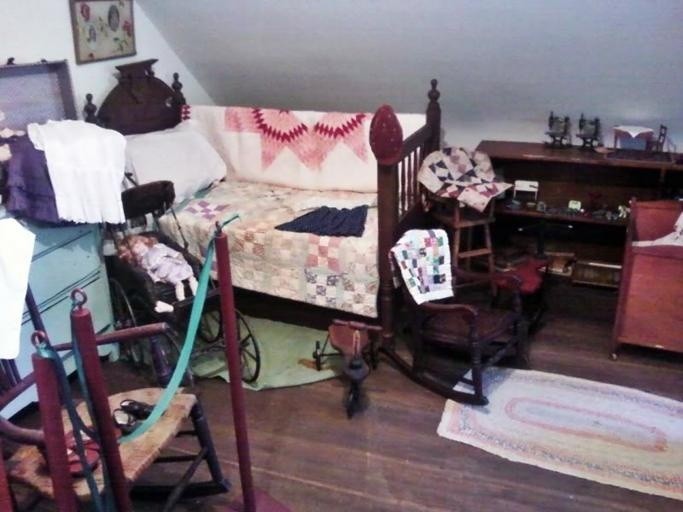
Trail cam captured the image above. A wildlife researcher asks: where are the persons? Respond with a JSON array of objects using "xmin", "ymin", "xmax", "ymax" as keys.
[{"xmin": 117, "ymin": 234, "xmax": 200, "ymax": 303}]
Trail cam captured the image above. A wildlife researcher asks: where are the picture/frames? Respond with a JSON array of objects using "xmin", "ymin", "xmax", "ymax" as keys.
[{"xmin": 68, "ymin": 0, "xmax": 136, "ymax": 66}]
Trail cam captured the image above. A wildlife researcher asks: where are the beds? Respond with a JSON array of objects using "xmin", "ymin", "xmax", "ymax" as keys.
[{"xmin": 85, "ymin": 60, "xmax": 441, "ymax": 347}]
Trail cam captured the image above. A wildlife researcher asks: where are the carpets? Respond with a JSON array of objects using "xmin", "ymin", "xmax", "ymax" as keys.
[{"xmin": 436, "ymin": 364, "xmax": 683, "ymax": 501}]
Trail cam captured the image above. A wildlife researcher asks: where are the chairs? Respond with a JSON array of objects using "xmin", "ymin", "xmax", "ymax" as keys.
[
  {"xmin": 377, "ymin": 226, "xmax": 533, "ymax": 402},
  {"xmin": 608, "ymin": 196, "xmax": 683, "ymax": 361},
  {"xmin": 419, "ymin": 146, "xmax": 501, "ymax": 279},
  {"xmin": 0, "ymin": 319, "xmax": 233, "ymax": 510}
]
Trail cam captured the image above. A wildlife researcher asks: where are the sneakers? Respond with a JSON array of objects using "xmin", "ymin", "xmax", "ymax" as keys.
[{"xmin": 113, "ymin": 399, "xmax": 165, "ymax": 433}]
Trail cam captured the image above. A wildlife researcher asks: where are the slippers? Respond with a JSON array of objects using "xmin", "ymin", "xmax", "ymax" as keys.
[{"xmin": 40, "ymin": 425, "xmax": 122, "ymax": 473}]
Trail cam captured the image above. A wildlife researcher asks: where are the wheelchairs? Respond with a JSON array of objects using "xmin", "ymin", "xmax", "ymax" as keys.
[{"xmin": 105, "ymin": 180, "xmax": 262, "ymax": 385}]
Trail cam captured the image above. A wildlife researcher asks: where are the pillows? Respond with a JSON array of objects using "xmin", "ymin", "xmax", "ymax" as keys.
[{"xmin": 126, "ymin": 122, "xmax": 229, "ymax": 212}]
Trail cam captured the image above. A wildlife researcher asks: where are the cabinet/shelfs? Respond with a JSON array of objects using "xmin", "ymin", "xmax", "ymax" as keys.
[
  {"xmin": 471, "ymin": 138, "xmax": 683, "ymax": 288},
  {"xmin": 0, "ymin": 201, "xmax": 125, "ymax": 429}
]
